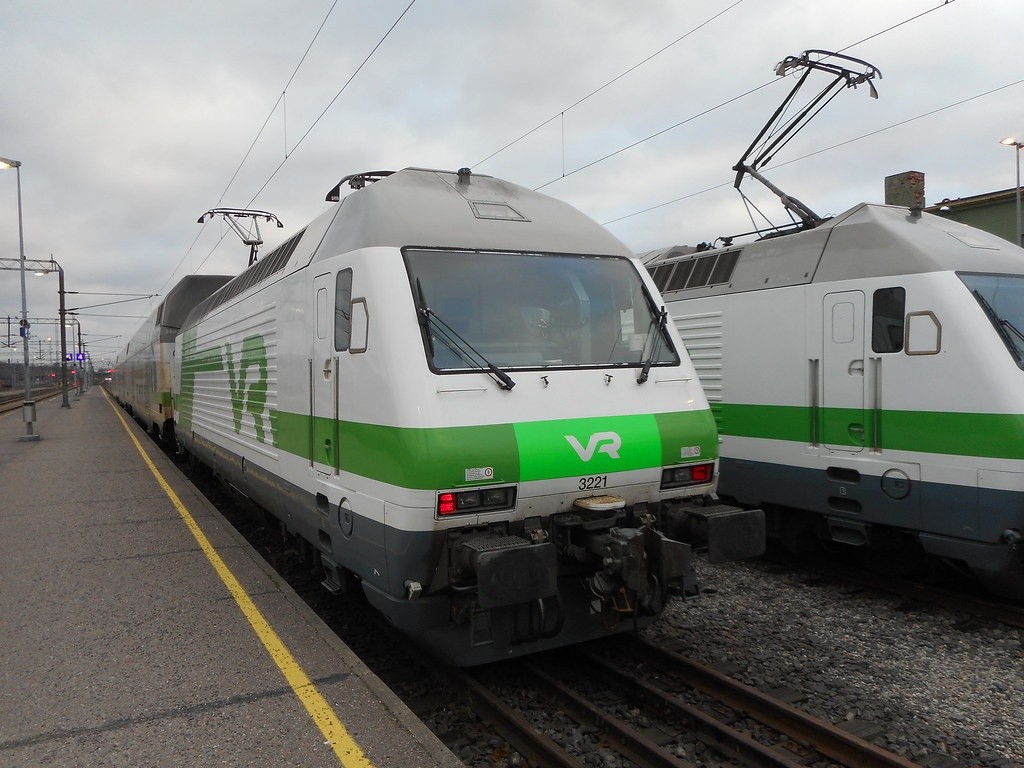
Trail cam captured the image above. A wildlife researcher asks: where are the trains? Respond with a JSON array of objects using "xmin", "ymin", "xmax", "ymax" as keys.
[
  {"xmin": 625, "ymin": 49, "xmax": 1024, "ymax": 595},
  {"xmin": 105, "ymin": 164, "xmax": 722, "ymax": 673}
]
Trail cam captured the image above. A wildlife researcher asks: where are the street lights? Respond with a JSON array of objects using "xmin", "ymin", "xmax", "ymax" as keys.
[
  {"xmin": 999, "ymin": 138, "xmax": 1024, "ymax": 249},
  {"xmin": 0, "ymin": 157, "xmax": 32, "ymax": 402},
  {"xmin": 63, "ymin": 324, "xmax": 76, "ymax": 368},
  {"xmin": 33, "ymin": 267, "xmax": 72, "ymax": 408}
]
